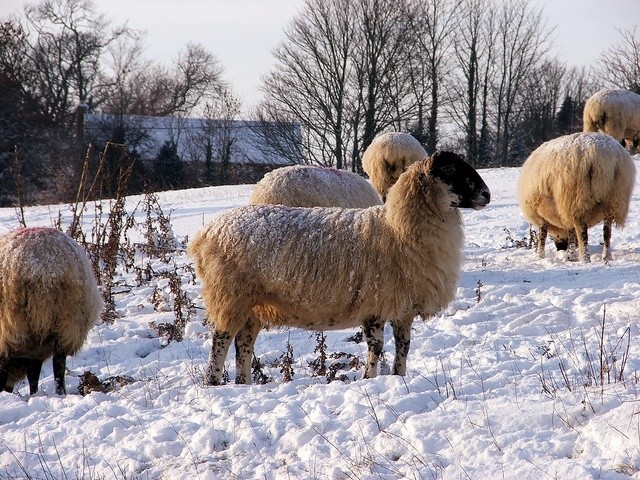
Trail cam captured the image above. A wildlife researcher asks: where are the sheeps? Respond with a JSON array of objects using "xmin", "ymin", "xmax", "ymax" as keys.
[
  {"xmin": 361, "ymin": 132, "xmax": 429, "ymax": 204},
  {"xmin": 0, "ymin": 226, "xmax": 100, "ymax": 396},
  {"xmin": 250, "ymin": 165, "xmax": 383, "ymax": 208},
  {"xmin": 191, "ymin": 152, "xmax": 490, "ymax": 386},
  {"xmin": 583, "ymin": 88, "xmax": 639, "ymax": 153},
  {"xmin": 519, "ymin": 132, "xmax": 635, "ymax": 263}
]
